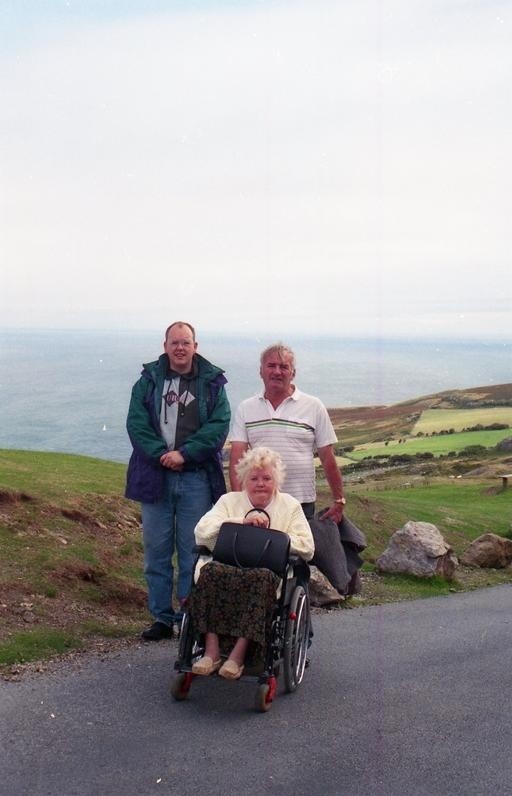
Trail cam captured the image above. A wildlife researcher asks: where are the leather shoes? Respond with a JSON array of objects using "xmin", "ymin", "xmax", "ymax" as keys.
[
  {"xmin": 142, "ymin": 622, "xmax": 174, "ymax": 640},
  {"xmin": 218, "ymin": 660, "xmax": 245, "ymax": 682},
  {"xmin": 191, "ymin": 656, "xmax": 222, "ymax": 677}
]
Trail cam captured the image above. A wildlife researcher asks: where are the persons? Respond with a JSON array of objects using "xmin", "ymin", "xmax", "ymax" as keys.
[
  {"xmin": 187, "ymin": 446, "xmax": 316, "ymax": 680},
  {"xmin": 124, "ymin": 320, "xmax": 231, "ymax": 642},
  {"xmin": 228, "ymin": 342, "xmax": 347, "ymax": 670}
]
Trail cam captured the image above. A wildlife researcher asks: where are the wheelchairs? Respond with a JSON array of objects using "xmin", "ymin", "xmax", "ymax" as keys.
[{"xmin": 170, "ymin": 546, "xmax": 311, "ymax": 713}]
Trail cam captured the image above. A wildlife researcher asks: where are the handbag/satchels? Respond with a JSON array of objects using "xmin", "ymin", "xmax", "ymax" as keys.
[{"xmin": 211, "ymin": 505, "xmax": 292, "ymax": 580}]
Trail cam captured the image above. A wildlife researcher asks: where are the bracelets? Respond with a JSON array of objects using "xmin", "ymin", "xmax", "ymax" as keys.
[{"xmin": 334, "ymin": 498, "xmax": 346, "ymax": 504}]
